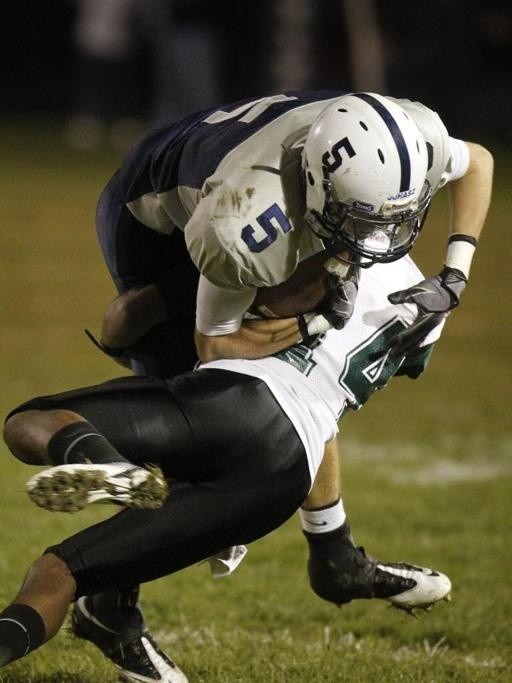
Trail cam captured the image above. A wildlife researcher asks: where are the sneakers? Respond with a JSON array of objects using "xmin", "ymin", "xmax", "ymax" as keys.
[
  {"xmin": 72, "ymin": 595, "xmax": 187, "ymax": 683},
  {"xmin": 307, "ymin": 558, "xmax": 453, "ymax": 609},
  {"xmin": 24, "ymin": 463, "xmax": 169, "ymax": 513}
]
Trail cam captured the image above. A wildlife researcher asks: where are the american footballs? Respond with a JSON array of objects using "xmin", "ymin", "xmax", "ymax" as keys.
[{"xmin": 248, "ymin": 243, "xmax": 351, "ymax": 320}]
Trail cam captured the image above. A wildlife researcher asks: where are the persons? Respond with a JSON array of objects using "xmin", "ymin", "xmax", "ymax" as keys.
[
  {"xmin": 83, "ymin": 91, "xmax": 495, "ymax": 682},
  {"xmin": 1, "ymin": 220, "xmax": 434, "ymax": 665}
]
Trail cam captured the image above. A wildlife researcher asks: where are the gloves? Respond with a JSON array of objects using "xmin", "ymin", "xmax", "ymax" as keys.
[
  {"xmin": 387, "ymin": 266, "xmax": 468, "ymax": 357},
  {"xmin": 324, "ymin": 253, "xmax": 360, "ymax": 331}
]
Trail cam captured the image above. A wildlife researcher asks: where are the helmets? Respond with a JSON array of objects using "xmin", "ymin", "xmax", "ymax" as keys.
[{"xmin": 301, "ymin": 93, "xmax": 429, "ymax": 269}]
